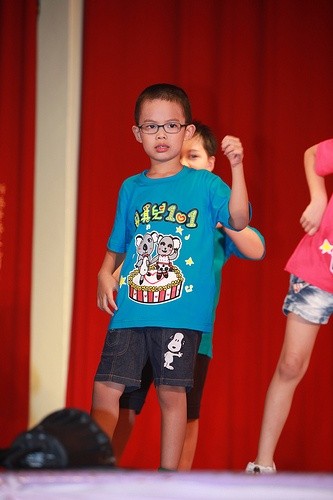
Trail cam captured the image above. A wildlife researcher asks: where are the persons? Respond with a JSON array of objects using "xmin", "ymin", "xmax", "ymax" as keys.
[
  {"xmin": 243, "ymin": 138, "xmax": 333, "ymax": 474},
  {"xmin": 89, "ymin": 83, "xmax": 254, "ymax": 473},
  {"xmin": 109, "ymin": 120, "xmax": 266, "ymax": 472}
]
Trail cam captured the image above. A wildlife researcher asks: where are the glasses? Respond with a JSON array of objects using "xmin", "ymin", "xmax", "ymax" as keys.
[{"xmin": 138, "ymin": 121, "xmax": 188, "ymax": 134}]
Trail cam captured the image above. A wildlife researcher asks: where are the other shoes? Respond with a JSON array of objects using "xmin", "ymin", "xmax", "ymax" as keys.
[{"xmin": 245, "ymin": 458, "xmax": 277, "ymax": 476}]
